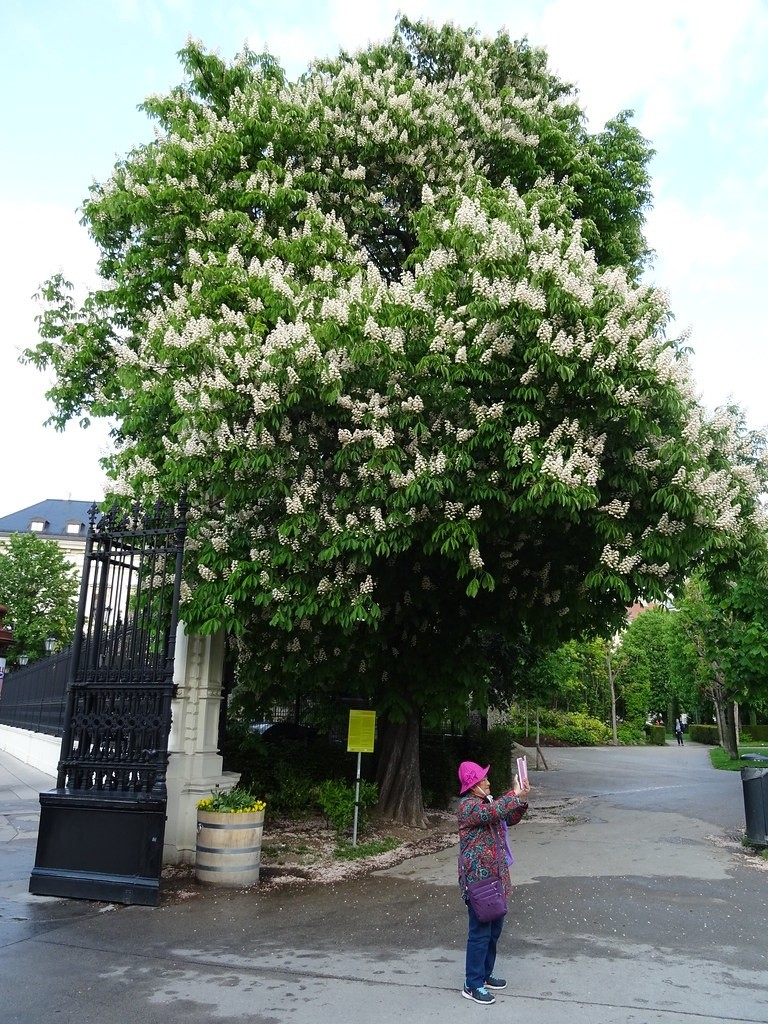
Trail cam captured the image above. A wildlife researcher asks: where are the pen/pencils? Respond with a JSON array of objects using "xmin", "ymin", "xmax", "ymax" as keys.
[{"xmin": 739, "ymin": 752, "xmax": 768, "ymax": 845}]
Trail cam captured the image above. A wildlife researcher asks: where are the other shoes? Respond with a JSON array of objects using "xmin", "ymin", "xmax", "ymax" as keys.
[
  {"xmin": 678, "ymin": 745, "xmax": 680, "ymax": 746},
  {"xmin": 682, "ymin": 744, "xmax": 684, "ymax": 746}
]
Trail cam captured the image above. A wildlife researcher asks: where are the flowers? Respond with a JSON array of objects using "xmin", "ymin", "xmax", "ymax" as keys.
[{"xmin": 195, "ymin": 781, "xmax": 266, "ymax": 813}]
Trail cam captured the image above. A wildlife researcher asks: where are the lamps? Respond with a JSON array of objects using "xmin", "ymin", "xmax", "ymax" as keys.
[
  {"xmin": 45, "ymin": 637, "xmax": 57, "ymax": 655},
  {"xmin": 19, "ymin": 652, "xmax": 30, "ymax": 667}
]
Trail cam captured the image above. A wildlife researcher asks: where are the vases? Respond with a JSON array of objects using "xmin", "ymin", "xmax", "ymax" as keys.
[{"xmin": 195, "ymin": 809, "xmax": 265, "ymax": 888}]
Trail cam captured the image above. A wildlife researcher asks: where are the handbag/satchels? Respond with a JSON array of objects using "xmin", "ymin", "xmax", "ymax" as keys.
[{"xmin": 468, "ymin": 877, "xmax": 507, "ymax": 923}]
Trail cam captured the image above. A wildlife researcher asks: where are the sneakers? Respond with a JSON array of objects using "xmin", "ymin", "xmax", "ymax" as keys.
[
  {"xmin": 483, "ymin": 972, "xmax": 507, "ymax": 989},
  {"xmin": 462, "ymin": 979, "xmax": 496, "ymax": 1005}
]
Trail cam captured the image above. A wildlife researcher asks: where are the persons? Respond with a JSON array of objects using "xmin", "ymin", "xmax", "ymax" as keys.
[
  {"xmin": 674, "ymin": 709, "xmax": 691, "ymax": 747},
  {"xmin": 457, "ymin": 762, "xmax": 530, "ymax": 1005},
  {"xmin": 655, "ymin": 720, "xmax": 660, "ymax": 726}
]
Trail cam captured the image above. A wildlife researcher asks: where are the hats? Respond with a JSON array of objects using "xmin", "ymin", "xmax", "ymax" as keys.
[{"xmin": 458, "ymin": 761, "xmax": 490, "ymax": 794}]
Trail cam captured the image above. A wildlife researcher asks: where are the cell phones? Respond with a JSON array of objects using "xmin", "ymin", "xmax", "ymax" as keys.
[{"xmin": 516, "ymin": 758, "xmax": 527, "ymax": 790}]
